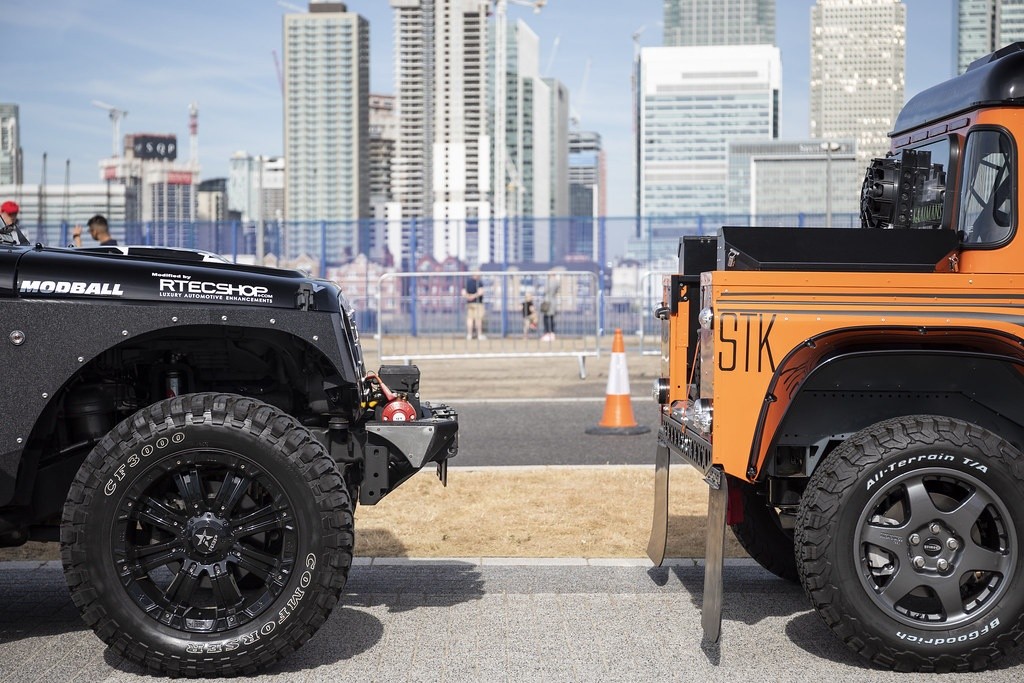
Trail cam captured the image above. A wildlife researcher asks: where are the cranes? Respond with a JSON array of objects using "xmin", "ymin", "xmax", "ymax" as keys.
[{"xmin": 92, "ymin": 99, "xmax": 129, "ymax": 158}]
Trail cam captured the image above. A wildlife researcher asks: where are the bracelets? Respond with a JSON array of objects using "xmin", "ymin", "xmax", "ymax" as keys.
[{"xmin": 72, "ymin": 233, "xmax": 80, "ymax": 239}]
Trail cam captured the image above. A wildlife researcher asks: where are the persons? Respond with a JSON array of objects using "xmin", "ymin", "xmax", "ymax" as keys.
[
  {"xmin": 461, "ymin": 272, "xmax": 487, "ymax": 341},
  {"xmin": 540, "ymin": 282, "xmax": 558, "ymax": 342},
  {"xmin": 518, "ymin": 292, "xmax": 538, "ymax": 340},
  {"xmin": 0, "ymin": 200, "xmax": 31, "ymax": 247},
  {"xmin": 72, "ymin": 214, "xmax": 118, "ymax": 246}
]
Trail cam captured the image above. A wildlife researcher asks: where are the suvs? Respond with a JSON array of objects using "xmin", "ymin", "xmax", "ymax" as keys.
[
  {"xmin": 644, "ymin": 42, "xmax": 1023, "ymax": 673},
  {"xmin": 0, "ymin": 212, "xmax": 461, "ymax": 679}
]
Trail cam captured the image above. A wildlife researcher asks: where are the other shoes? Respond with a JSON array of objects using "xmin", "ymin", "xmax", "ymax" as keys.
[
  {"xmin": 478, "ymin": 335, "xmax": 486, "ymax": 341},
  {"xmin": 467, "ymin": 336, "xmax": 473, "ymax": 340},
  {"xmin": 540, "ymin": 332, "xmax": 556, "ymax": 341}
]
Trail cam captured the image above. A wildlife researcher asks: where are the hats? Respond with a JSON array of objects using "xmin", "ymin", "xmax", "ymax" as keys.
[{"xmin": 1, "ymin": 201, "xmax": 19, "ymax": 214}]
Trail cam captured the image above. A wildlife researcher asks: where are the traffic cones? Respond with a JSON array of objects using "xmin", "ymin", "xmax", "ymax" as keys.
[{"xmin": 584, "ymin": 326, "xmax": 651, "ymax": 435}]
